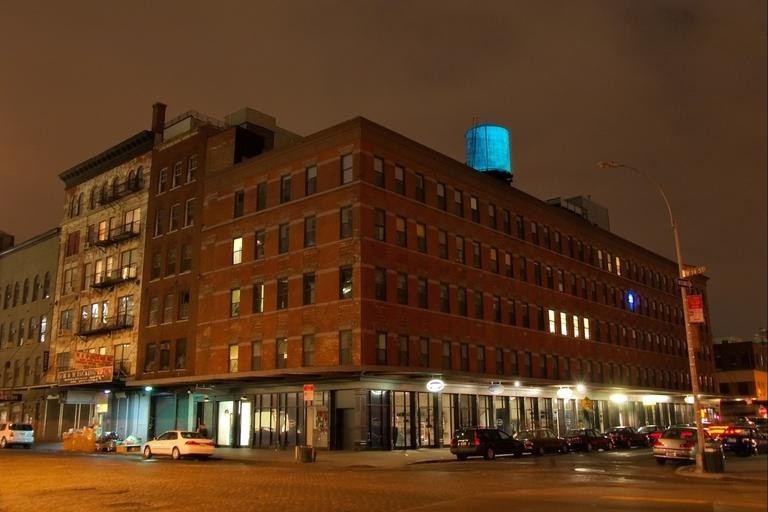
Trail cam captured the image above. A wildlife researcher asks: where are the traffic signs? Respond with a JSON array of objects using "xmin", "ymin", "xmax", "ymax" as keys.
[{"xmin": 674, "ymin": 264, "xmax": 708, "ymax": 289}]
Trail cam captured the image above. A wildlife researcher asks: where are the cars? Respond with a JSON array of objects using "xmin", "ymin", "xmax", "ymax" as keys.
[{"xmin": 140, "ymin": 430, "xmax": 216, "ymax": 460}]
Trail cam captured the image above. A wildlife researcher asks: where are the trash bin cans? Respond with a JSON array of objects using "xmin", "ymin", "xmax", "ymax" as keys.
[{"xmin": 704, "ymin": 445, "xmax": 724, "ymax": 473}]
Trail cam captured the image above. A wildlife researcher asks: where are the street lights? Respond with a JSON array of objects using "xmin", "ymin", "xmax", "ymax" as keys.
[{"xmin": 596, "ymin": 160, "xmax": 725, "ymax": 474}]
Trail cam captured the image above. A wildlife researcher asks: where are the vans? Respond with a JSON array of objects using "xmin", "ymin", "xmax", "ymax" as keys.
[{"xmin": 0, "ymin": 422, "xmax": 35, "ymax": 449}]
